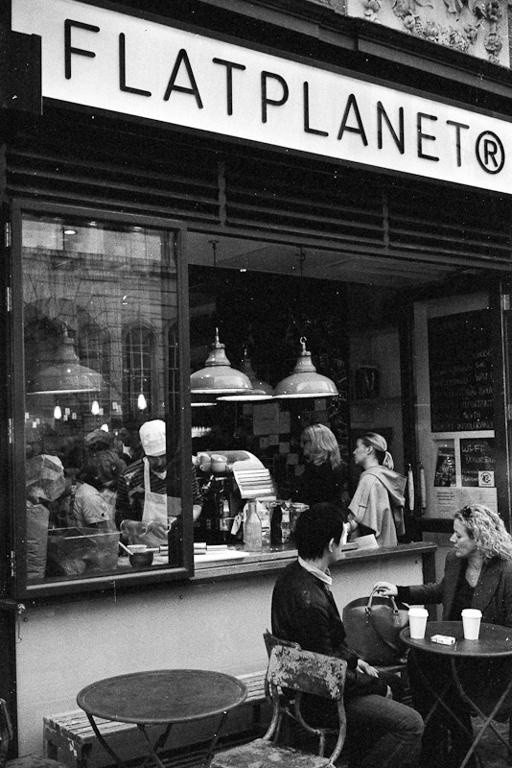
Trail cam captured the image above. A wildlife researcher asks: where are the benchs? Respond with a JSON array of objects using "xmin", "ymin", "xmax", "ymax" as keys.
[{"xmin": 42, "ymin": 643, "xmax": 415, "ymax": 768}]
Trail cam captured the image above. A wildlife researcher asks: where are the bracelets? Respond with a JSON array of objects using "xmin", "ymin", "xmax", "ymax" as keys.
[{"xmin": 348, "ymin": 520, "xmax": 354, "ymax": 530}]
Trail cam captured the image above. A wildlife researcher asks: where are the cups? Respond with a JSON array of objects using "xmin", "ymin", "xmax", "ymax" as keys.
[
  {"xmin": 408, "ymin": 609, "xmax": 428, "ymax": 639},
  {"xmin": 461, "ymin": 609, "xmax": 482, "ymax": 639},
  {"xmin": 199, "ymin": 454, "xmax": 227, "ymax": 472}
]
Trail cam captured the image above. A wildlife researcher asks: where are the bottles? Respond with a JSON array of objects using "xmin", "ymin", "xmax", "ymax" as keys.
[
  {"xmin": 243, "ymin": 503, "xmax": 262, "ymax": 551},
  {"xmin": 219, "ymin": 500, "xmax": 234, "ymax": 542},
  {"xmin": 270, "ymin": 503, "xmax": 282, "ymax": 551},
  {"xmin": 432, "ymin": 400, "xmax": 494, "ymax": 432}
]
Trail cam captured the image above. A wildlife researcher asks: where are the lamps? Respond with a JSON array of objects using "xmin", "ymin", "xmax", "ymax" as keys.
[
  {"xmin": 89, "ymin": 396, "xmax": 104, "ymax": 418},
  {"xmin": 214, "ymin": 255, "xmax": 274, "ymax": 401},
  {"xmin": 53, "ymin": 399, "xmax": 66, "ymax": 422},
  {"xmin": 189, "ymin": 236, "xmax": 253, "ymax": 393},
  {"xmin": 277, "ymin": 240, "xmax": 341, "ymax": 398},
  {"xmin": 135, "ymin": 225, "xmax": 149, "ymax": 410},
  {"xmin": 26, "ymin": 225, "xmax": 103, "ymax": 395}
]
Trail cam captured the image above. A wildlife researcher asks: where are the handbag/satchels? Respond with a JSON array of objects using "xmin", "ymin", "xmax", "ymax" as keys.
[{"xmin": 342, "ymin": 585, "xmax": 412, "ymax": 666}]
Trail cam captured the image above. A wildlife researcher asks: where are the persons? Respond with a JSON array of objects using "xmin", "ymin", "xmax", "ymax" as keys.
[
  {"xmin": 292, "ymin": 423, "xmax": 351, "ymax": 518},
  {"xmin": 343, "ymin": 431, "xmax": 408, "ymax": 547},
  {"xmin": 270, "ymin": 502, "xmax": 426, "ymax": 767},
  {"xmin": 24, "ymin": 418, "xmax": 204, "ymax": 547},
  {"xmin": 374, "ymin": 503, "xmax": 512, "ymax": 768}
]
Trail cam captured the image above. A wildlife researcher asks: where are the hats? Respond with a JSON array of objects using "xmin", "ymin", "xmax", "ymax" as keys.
[{"xmin": 138, "ymin": 419, "xmax": 167, "ymax": 458}]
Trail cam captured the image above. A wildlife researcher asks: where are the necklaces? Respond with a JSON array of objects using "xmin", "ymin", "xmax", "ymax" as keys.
[{"xmin": 467, "ymin": 567, "xmax": 478, "ymax": 580}]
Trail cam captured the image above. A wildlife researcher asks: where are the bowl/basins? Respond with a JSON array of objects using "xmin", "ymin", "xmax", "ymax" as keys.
[{"xmin": 127, "ymin": 545, "xmax": 147, "ymax": 553}]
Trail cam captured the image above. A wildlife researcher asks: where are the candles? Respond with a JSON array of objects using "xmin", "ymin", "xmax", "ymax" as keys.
[
  {"xmin": 419, "ymin": 460, "xmax": 426, "ymax": 511},
  {"xmin": 407, "ymin": 463, "xmax": 415, "ymax": 511}
]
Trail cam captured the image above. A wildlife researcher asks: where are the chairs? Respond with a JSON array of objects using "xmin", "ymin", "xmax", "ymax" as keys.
[
  {"xmin": 210, "ymin": 647, "xmax": 352, "ymax": 767},
  {"xmin": 262, "ymin": 632, "xmax": 350, "ymax": 758}
]
